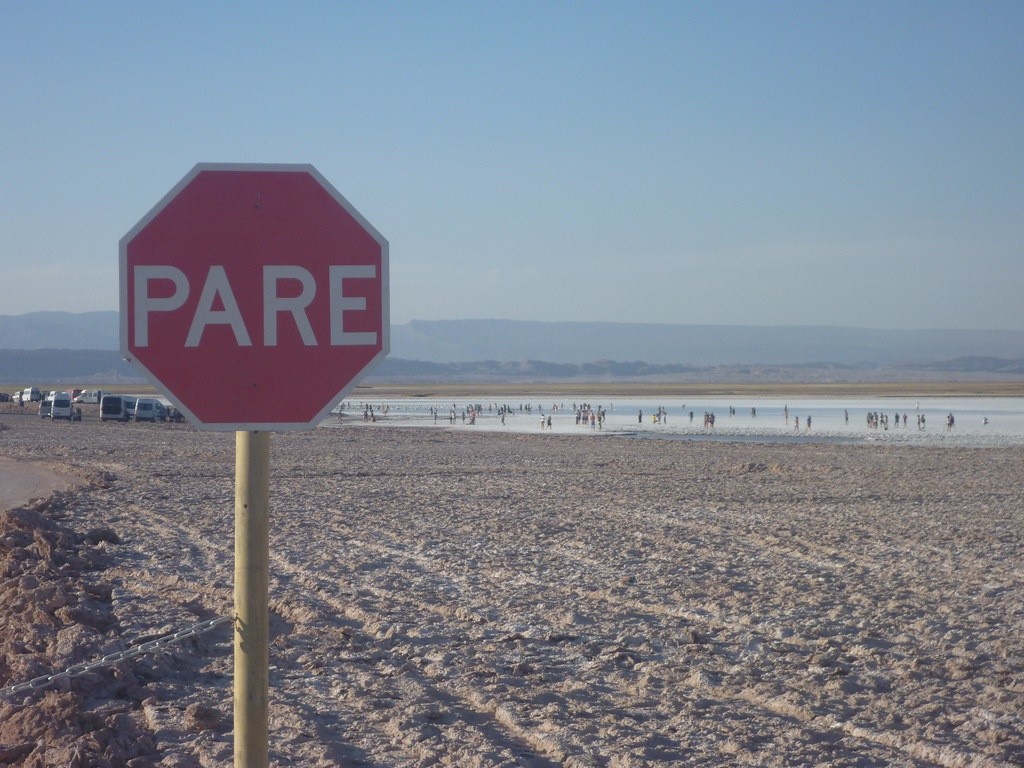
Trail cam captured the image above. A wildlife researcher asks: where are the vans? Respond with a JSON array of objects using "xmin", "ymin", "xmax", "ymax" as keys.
[{"xmin": 0, "ymin": 386, "xmax": 187, "ymax": 424}]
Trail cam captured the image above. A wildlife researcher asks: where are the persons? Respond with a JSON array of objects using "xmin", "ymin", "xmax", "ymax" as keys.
[{"xmin": 0, "ymin": 386, "xmax": 1021, "ymax": 438}]
